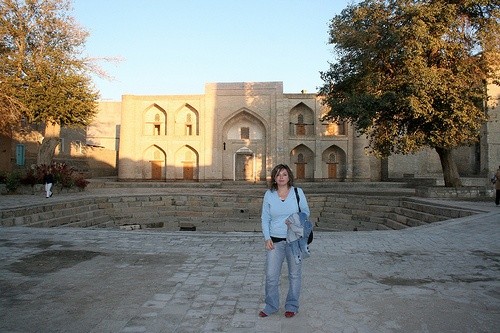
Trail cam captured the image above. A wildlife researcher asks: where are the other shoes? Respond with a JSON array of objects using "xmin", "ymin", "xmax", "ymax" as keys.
[{"xmin": 45, "ymin": 192, "xmax": 54, "ymax": 199}]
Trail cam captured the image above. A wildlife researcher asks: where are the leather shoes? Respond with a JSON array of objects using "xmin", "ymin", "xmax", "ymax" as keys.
[
  {"xmin": 285, "ymin": 311, "xmax": 296, "ymax": 318},
  {"xmin": 259, "ymin": 312, "xmax": 268, "ymax": 317}
]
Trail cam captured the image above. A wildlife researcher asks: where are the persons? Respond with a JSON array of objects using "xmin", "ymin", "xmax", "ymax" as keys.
[
  {"xmin": 43, "ymin": 170, "xmax": 54, "ymax": 198},
  {"xmin": 260, "ymin": 164, "xmax": 313, "ymax": 318}
]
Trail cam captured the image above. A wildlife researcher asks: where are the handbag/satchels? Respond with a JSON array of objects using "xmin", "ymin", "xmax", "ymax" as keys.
[{"xmin": 294, "ymin": 187, "xmax": 313, "ymax": 245}]
